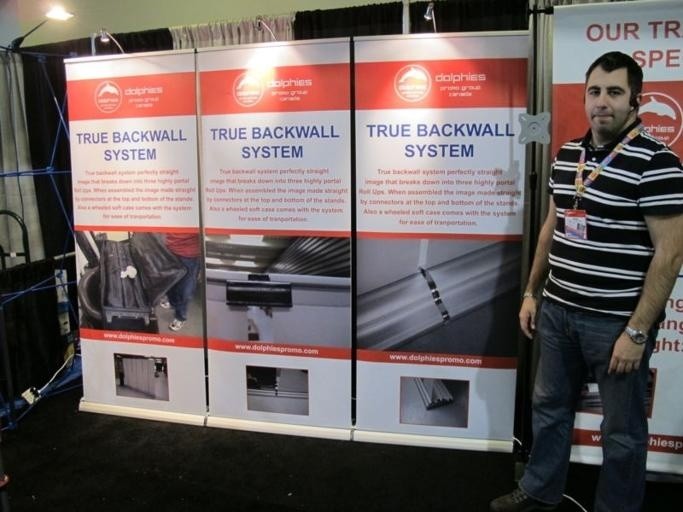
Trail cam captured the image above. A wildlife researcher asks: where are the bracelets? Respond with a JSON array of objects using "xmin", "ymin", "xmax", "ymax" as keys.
[{"xmin": 522, "ymin": 293, "xmax": 539, "ymax": 298}]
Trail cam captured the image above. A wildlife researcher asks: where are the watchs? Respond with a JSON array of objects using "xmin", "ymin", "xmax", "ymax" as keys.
[{"xmin": 624, "ymin": 326, "xmax": 649, "ymax": 344}]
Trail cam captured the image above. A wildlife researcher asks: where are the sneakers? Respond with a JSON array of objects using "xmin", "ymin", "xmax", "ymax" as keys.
[
  {"xmin": 488, "ymin": 487, "xmax": 556, "ymax": 512},
  {"xmin": 168, "ymin": 318, "xmax": 184, "ymax": 331},
  {"xmin": 159, "ymin": 299, "xmax": 171, "ymax": 309}
]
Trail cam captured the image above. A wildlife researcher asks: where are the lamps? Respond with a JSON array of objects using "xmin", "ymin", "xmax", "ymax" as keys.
[
  {"xmin": 11, "ymin": 8, "xmax": 74, "ymax": 50},
  {"xmin": 422, "ymin": 0, "xmax": 440, "ymax": 33},
  {"xmin": 100, "ymin": 29, "xmax": 124, "ymax": 55},
  {"xmin": 253, "ymin": 15, "xmax": 279, "ymax": 43}
]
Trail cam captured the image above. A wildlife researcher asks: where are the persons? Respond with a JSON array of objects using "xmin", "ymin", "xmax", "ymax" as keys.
[
  {"xmin": 157, "ymin": 230, "xmax": 202, "ymax": 331},
  {"xmin": 488, "ymin": 48, "xmax": 681, "ymax": 512}
]
